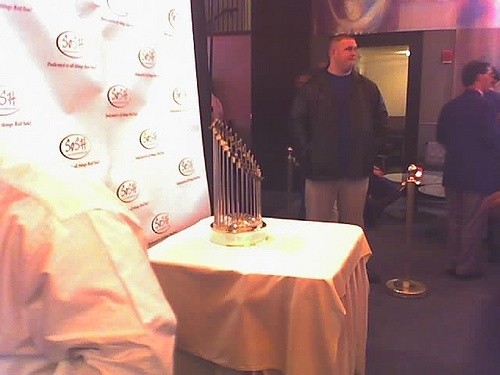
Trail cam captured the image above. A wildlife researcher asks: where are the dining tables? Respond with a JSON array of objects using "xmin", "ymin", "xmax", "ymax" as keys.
[{"xmin": 147, "ymin": 215, "xmax": 374, "ymax": 375}]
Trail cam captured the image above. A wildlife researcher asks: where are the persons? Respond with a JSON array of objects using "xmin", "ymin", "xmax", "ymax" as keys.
[
  {"xmin": 1, "ymin": 145, "xmax": 177, "ymax": 375},
  {"xmin": 486, "ymin": 70, "xmax": 499, "ymax": 262},
  {"xmin": 296, "ymin": 71, "xmax": 312, "ymax": 91},
  {"xmin": 437, "ymin": 61, "xmax": 500, "ymax": 277},
  {"xmin": 289, "ymin": 33, "xmax": 392, "ymax": 230}
]
